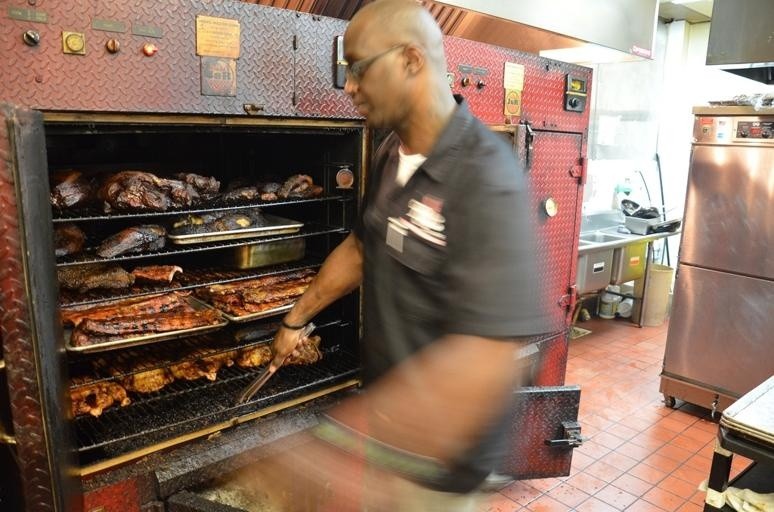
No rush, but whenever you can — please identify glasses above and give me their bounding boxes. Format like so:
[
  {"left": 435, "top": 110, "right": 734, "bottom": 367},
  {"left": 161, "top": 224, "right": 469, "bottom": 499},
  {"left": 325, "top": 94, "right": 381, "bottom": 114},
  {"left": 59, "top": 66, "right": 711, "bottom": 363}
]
[{"left": 343, "top": 44, "right": 410, "bottom": 79}]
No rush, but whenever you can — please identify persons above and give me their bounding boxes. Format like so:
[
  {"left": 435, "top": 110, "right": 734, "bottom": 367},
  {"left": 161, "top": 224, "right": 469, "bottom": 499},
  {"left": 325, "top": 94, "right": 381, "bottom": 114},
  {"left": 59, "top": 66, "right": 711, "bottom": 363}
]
[{"left": 244, "top": 1, "right": 555, "bottom": 512}]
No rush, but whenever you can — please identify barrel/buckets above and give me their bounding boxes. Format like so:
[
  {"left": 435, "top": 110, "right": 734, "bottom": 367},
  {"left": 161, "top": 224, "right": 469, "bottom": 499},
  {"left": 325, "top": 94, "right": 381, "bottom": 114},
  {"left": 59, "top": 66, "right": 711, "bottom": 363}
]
[
  {"left": 598, "top": 285, "right": 620, "bottom": 319},
  {"left": 631, "top": 264, "right": 672, "bottom": 328}
]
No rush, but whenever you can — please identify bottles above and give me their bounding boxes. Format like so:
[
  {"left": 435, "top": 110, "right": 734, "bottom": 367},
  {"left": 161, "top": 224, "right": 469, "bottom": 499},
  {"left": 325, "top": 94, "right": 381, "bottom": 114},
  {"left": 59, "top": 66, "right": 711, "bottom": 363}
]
[
  {"left": 598, "top": 280, "right": 620, "bottom": 319},
  {"left": 581, "top": 308, "right": 590, "bottom": 323}
]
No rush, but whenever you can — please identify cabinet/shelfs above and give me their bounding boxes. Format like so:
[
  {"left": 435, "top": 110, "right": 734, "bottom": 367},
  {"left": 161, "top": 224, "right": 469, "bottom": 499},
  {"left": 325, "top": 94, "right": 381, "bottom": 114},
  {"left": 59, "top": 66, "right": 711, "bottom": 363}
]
[{"left": 703, "top": 427, "right": 774, "bottom": 512}]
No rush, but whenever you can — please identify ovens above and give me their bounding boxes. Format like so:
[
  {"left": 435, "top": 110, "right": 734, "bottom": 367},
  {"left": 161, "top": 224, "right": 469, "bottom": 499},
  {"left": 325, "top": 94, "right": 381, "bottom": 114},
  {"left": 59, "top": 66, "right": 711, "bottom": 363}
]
[{"left": 2, "top": 2, "right": 594, "bottom": 510}]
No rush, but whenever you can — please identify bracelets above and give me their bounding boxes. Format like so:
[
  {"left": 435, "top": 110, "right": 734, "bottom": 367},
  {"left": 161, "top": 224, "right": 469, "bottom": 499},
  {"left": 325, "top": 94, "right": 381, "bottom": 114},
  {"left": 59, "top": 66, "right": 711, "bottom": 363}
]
[{"left": 282, "top": 319, "right": 308, "bottom": 330}]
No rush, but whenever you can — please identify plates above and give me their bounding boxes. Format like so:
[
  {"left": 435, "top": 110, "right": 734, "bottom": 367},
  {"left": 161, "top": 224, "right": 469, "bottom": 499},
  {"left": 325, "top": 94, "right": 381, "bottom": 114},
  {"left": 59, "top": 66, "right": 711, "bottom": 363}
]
[
  {"left": 166, "top": 214, "right": 305, "bottom": 246},
  {"left": 220, "top": 301, "right": 300, "bottom": 326},
  {"left": 63, "top": 293, "right": 228, "bottom": 352}
]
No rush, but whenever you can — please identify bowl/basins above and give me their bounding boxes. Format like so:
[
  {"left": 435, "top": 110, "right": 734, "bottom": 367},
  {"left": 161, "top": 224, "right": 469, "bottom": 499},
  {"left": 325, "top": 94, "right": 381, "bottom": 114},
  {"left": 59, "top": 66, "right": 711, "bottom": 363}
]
[{"left": 620, "top": 199, "right": 644, "bottom": 218}]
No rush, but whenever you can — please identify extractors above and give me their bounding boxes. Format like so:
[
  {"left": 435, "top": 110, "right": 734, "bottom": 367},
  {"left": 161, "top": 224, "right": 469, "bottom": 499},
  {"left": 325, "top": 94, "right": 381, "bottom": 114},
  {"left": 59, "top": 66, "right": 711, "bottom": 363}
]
[{"left": 443, "top": 1, "right": 666, "bottom": 60}]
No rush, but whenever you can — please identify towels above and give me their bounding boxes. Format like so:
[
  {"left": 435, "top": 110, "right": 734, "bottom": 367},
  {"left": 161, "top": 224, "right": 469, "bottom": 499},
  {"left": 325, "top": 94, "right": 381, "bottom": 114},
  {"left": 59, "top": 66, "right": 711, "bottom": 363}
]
[{"left": 698, "top": 478, "right": 774, "bottom": 511}]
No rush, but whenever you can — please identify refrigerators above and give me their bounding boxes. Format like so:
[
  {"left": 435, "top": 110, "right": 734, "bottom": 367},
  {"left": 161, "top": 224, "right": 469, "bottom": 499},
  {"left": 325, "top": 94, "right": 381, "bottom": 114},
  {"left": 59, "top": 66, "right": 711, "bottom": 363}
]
[{"left": 660, "top": 106, "right": 773, "bottom": 415}]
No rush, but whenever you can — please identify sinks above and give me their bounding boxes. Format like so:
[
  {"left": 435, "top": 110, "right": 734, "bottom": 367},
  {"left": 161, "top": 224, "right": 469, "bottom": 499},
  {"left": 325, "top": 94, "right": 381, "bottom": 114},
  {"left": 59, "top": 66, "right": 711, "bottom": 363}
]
[
  {"left": 576, "top": 241, "right": 615, "bottom": 295},
  {"left": 580, "top": 233, "right": 647, "bottom": 283}
]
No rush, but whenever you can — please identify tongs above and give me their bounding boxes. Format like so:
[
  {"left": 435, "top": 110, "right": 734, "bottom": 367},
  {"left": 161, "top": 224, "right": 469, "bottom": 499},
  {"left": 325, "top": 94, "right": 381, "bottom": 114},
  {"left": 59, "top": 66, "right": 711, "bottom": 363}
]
[{"left": 234, "top": 322, "right": 317, "bottom": 406}]
